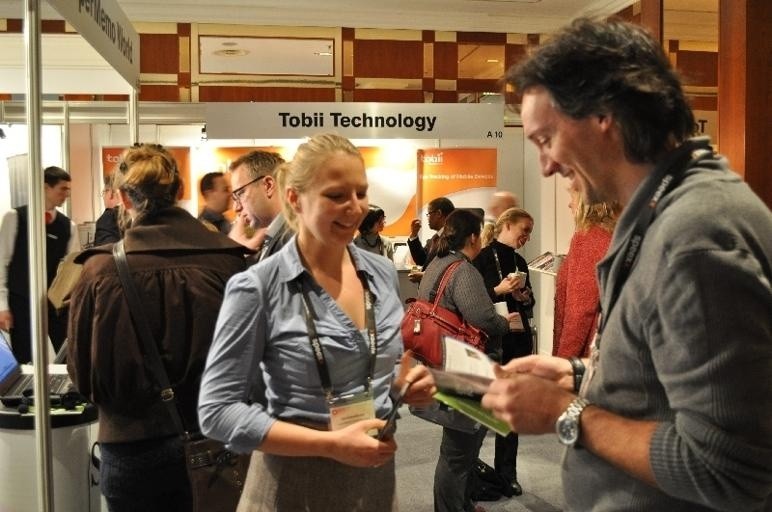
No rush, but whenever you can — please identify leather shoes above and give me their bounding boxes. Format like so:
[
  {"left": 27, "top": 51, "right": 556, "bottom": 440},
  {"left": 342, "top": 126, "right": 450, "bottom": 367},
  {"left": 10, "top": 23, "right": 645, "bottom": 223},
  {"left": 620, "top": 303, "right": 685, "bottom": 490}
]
[{"left": 505, "top": 480, "right": 523, "bottom": 494}]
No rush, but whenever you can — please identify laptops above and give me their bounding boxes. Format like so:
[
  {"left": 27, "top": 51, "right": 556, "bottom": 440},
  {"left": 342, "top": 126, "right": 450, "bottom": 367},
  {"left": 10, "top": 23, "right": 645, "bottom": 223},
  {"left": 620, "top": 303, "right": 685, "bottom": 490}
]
[{"left": 0, "top": 326, "right": 72, "bottom": 405}]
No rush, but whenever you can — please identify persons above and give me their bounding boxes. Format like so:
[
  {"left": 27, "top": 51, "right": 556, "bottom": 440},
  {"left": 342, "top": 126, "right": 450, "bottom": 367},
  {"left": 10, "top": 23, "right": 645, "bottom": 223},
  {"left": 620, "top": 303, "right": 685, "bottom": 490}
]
[
  {"left": 90, "top": 169, "right": 132, "bottom": 248},
  {"left": 195, "top": 171, "right": 521, "bottom": 301},
  {"left": 473, "top": 206, "right": 537, "bottom": 504},
  {"left": 65, "top": 143, "right": 268, "bottom": 512},
  {"left": 479, "top": 12, "right": 771, "bottom": 510},
  {"left": 0, "top": 165, "right": 79, "bottom": 365},
  {"left": 415, "top": 207, "right": 522, "bottom": 511},
  {"left": 195, "top": 132, "right": 439, "bottom": 512},
  {"left": 551, "top": 173, "right": 619, "bottom": 361},
  {"left": 227, "top": 149, "right": 297, "bottom": 267}
]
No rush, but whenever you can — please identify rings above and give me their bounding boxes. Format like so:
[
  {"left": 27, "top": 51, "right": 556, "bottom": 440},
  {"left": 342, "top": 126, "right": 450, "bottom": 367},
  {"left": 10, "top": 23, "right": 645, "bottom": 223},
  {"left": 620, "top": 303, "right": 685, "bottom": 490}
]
[{"left": 372, "top": 464, "right": 379, "bottom": 468}]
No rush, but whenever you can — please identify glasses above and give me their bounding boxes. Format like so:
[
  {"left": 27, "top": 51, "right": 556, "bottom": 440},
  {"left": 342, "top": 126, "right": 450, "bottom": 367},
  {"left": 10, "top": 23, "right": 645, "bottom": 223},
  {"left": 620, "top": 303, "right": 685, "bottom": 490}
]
[{"left": 231, "top": 177, "right": 266, "bottom": 201}]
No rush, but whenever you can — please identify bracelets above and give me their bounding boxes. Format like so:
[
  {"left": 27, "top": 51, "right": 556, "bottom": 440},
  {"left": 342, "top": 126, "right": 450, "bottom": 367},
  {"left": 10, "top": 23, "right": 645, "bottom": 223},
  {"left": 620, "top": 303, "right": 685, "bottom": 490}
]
[{"left": 564, "top": 354, "right": 587, "bottom": 392}]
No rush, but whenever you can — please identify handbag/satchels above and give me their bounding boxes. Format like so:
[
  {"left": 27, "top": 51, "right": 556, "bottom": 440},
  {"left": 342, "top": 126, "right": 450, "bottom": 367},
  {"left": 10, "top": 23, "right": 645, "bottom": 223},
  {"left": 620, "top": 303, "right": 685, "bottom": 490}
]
[
  {"left": 472, "top": 457, "right": 501, "bottom": 502},
  {"left": 409, "top": 399, "right": 481, "bottom": 433},
  {"left": 178, "top": 431, "right": 252, "bottom": 512},
  {"left": 403, "top": 300, "right": 487, "bottom": 368}
]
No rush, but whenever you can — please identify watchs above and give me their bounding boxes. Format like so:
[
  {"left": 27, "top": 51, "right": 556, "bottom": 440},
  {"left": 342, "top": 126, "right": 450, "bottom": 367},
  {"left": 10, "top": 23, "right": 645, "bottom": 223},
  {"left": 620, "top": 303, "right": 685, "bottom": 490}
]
[{"left": 554, "top": 396, "right": 594, "bottom": 452}]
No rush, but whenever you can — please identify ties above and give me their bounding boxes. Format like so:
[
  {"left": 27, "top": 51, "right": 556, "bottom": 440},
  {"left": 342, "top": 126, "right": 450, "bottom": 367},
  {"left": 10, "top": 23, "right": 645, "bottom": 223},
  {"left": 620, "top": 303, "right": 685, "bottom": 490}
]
[{"left": 45, "top": 212, "right": 52, "bottom": 223}]
